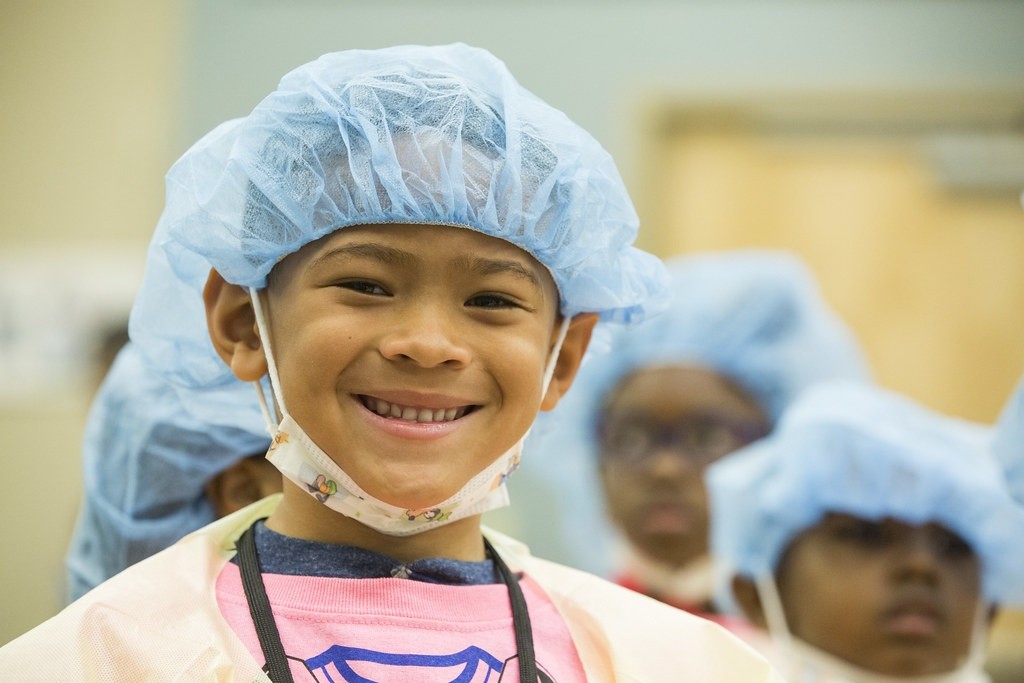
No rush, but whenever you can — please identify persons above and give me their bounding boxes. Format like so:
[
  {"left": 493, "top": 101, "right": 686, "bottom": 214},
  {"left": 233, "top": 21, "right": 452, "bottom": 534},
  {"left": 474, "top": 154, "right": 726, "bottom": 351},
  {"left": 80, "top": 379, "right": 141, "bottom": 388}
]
[
  {"left": 730, "top": 407, "right": 1019, "bottom": 680},
  {"left": 0, "top": 65, "right": 778, "bottom": 683},
  {"left": 83, "top": 358, "right": 293, "bottom": 530},
  {"left": 560, "top": 281, "right": 830, "bottom": 568}
]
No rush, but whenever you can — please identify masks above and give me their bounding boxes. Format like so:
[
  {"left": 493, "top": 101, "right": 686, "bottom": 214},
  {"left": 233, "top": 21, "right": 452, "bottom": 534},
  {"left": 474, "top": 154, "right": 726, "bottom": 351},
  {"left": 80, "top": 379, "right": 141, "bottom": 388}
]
[
  {"left": 245, "top": 285, "right": 571, "bottom": 539},
  {"left": 749, "top": 571, "right": 992, "bottom": 683}
]
